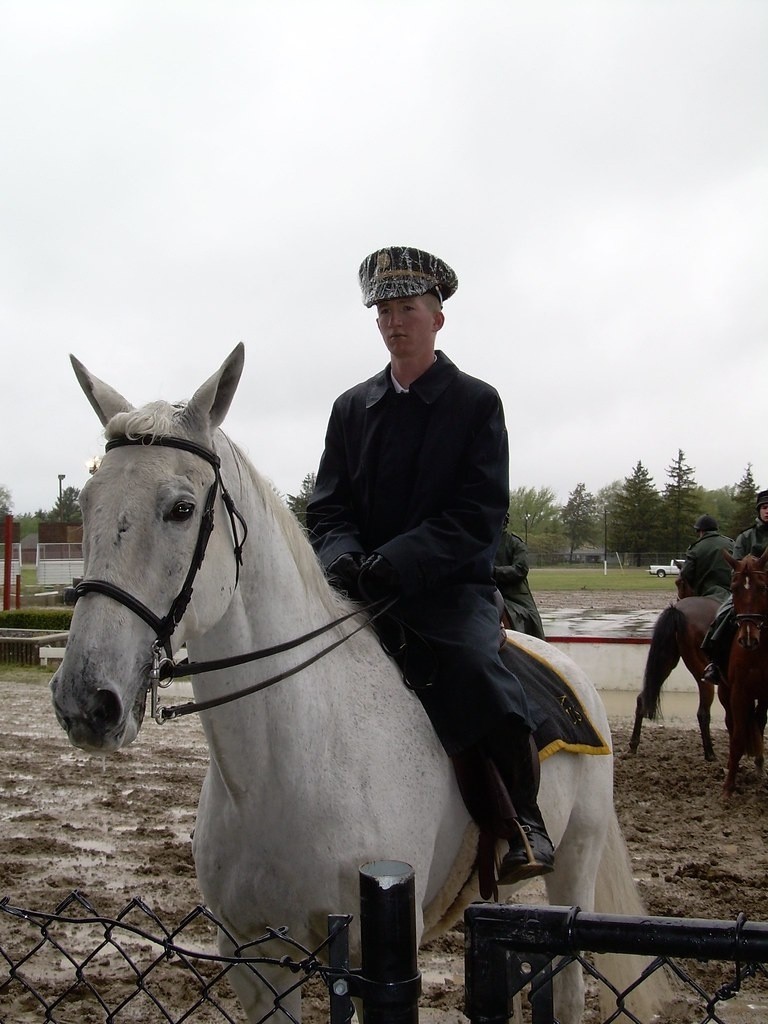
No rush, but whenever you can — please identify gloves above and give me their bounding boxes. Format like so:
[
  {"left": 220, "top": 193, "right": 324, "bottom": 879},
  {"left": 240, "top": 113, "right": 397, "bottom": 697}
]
[{"left": 328, "top": 552, "right": 391, "bottom": 595}]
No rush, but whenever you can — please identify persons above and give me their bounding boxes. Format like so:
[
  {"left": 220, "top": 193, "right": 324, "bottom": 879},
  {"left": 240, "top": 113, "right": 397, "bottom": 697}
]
[
  {"left": 305, "top": 247, "right": 557, "bottom": 884},
  {"left": 680, "top": 490, "right": 768, "bottom": 685}
]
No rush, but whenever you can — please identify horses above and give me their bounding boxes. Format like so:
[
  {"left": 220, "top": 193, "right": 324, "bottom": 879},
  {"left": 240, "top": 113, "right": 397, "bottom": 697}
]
[
  {"left": 48, "top": 341, "right": 688, "bottom": 1024},
  {"left": 624, "top": 544, "right": 768, "bottom": 801}
]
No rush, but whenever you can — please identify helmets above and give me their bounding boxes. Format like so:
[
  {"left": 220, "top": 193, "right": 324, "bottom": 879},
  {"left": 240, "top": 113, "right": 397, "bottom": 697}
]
[
  {"left": 756, "top": 490, "right": 768, "bottom": 510},
  {"left": 693, "top": 514, "right": 720, "bottom": 532}
]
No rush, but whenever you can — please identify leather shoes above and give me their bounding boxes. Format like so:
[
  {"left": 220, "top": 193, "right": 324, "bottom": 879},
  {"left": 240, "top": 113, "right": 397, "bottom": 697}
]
[
  {"left": 501, "top": 833, "right": 559, "bottom": 873},
  {"left": 704, "top": 666, "right": 719, "bottom": 683}
]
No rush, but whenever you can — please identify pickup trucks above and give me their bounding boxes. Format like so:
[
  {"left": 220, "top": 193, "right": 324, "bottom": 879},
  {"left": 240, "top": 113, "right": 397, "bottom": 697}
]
[{"left": 649, "top": 559, "right": 686, "bottom": 578}]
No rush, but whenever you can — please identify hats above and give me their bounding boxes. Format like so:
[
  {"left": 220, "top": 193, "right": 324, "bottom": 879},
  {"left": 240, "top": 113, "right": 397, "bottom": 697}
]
[{"left": 358, "top": 246, "right": 458, "bottom": 308}]
